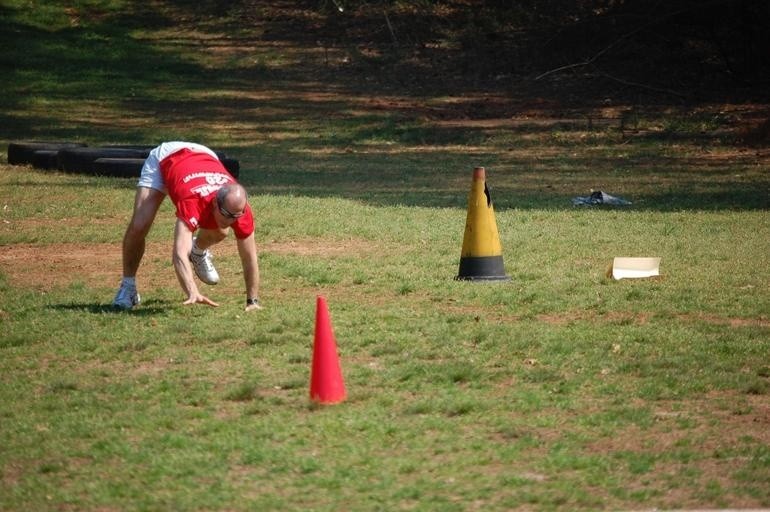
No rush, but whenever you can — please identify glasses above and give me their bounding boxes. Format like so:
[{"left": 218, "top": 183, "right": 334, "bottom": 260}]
[{"left": 217, "top": 199, "right": 245, "bottom": 218}]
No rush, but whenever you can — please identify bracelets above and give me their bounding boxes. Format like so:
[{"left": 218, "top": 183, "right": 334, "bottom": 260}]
[{"left": 247, "top": 298, "right": 257, "bottom": 304}]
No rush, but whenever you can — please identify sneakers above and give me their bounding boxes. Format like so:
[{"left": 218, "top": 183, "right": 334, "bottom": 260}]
[
  {"left": 189, "top": 237, "right": 219, "bottom": 285},
  {"left": 113, "top": 280, "right": 140, "bottom": 308}
]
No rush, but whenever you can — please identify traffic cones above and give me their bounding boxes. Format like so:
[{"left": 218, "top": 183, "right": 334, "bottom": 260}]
[
  {"left": 454, "top": 166, "right": 511, "bottom": 281},
  {"left": 309, "top": 295, "right": 347, "bottom": 404}
]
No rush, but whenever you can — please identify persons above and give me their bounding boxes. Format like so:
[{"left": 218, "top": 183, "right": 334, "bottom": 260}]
[{"left": 112, "top": 141, "right": 264, "bottom": 311}]
